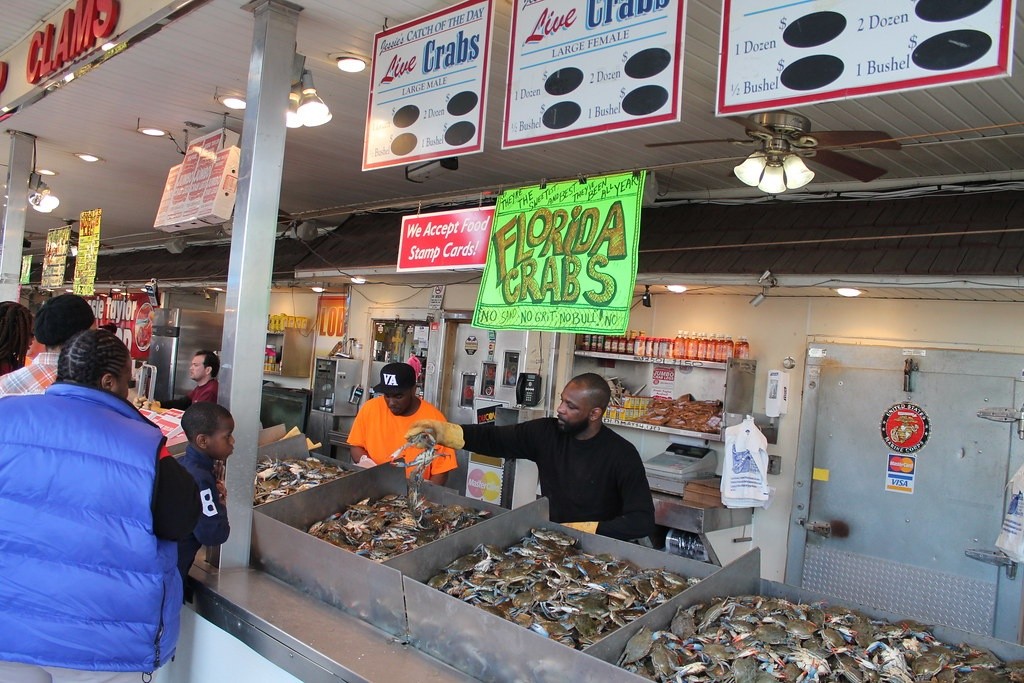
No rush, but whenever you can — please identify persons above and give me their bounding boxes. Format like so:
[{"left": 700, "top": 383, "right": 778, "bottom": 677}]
[
  {"left": 346, "top": 363, "right": 459, "bottom": 485},
  {"left": 0, "top": 301, "right": 34, "bottom": 375},
  {"left": 0, "top": 330, "right": 202, "bottom": 683},
  {"left": 175, "top": 403, "right": 235, "bottom": 579},
  {"left": 99, "top": 323, "right": 117, "bottom": 334},
  {"left": 0, "top": 295, "right": 96, "bottom": 395},
  {"left": 405, "top": 372, "right": 656, "bottom": 542},
  {"left": 133, "top": 350, "right": 220, "bottom": 410}
]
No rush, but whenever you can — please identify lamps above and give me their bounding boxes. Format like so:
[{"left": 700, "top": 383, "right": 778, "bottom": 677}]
[
  {"left": 28, "top": 185, "right": 59, "bottom": 214},
  {"left": 734, "top": 138, "right": 815, "bottom": 194},
  {"left": 286, "top": 69, "right": 333, "bottom": 129}
]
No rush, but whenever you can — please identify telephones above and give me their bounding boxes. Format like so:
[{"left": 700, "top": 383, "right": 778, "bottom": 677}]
[{"left": 516, "top": 372, "right": 542, "bottom": 406}]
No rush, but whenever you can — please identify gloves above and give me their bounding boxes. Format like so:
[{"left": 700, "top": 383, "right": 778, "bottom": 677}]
[
  {"left": 404, "top": 419, "right": 466, "bottom": 450},
  {"left": 560, "top": 521, "right": 599, "bottom": 533}
]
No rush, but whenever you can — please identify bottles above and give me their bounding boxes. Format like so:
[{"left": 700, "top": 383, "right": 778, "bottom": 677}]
[
  {"left": 355, "top": 338, "right": 363, "bottom": 360},
  {"left": 582, "top": 329, "right": 750, "bottom": 363}
]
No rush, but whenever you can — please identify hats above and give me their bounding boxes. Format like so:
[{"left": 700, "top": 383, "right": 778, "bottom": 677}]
[
  {"left": 373, "top": 362, "right": 416, "bottom": 394},
  {"left": 33, "top": 294, "right": 94, "bottom": 344},
  {"left": 99, "top": 323, "right": 116, "bottom": 334}
]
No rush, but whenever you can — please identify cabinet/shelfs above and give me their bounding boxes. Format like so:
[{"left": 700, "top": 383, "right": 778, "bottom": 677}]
[{"left": 573, "top": 351, "right": 757, "bottom": 441}]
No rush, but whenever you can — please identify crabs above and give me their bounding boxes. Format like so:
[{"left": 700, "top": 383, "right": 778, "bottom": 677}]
[{"left": 216, "top": 432, "right": 1024, "bottom": 683}]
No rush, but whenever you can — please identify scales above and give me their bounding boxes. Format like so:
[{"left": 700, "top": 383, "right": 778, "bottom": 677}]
[{"left": 643, "top": 443, "right": 718, "bottom": 496}]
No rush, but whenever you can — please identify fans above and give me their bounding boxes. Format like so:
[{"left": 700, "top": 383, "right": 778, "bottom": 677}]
[{"left": 644, "top": 111, "right": 901, "bottom": 196}]
